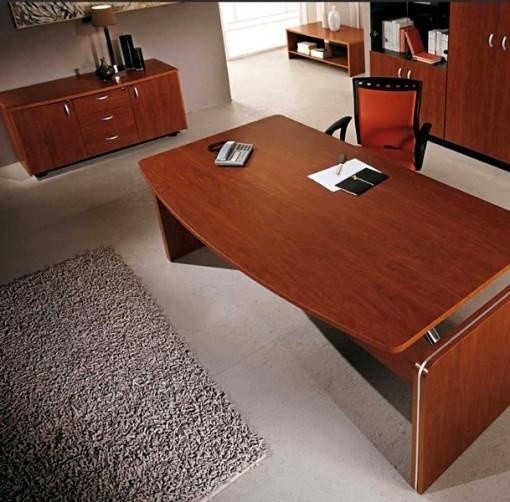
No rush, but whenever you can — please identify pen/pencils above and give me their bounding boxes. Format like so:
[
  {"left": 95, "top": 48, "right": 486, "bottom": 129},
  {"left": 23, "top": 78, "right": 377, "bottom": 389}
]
[{"left": 336, "top": 164, "right": 343, "bottom": 175}]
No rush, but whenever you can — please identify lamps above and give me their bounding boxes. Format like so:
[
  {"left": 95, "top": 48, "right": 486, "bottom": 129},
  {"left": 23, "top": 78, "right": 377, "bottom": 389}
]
[{"left": 92, "top": 4, "right": 127, "bottom": 79}]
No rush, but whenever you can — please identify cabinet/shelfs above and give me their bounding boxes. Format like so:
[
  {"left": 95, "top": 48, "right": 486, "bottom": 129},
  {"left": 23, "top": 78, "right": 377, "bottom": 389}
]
[
  {"left": 286, "top": 29, "right": 365, "bottom": 77},
  {"left": 370, "top": 0, "right": 450, "bottom": 147},
  {"left": 0, "top": 70, "right": 188, "bottom": 178},
  {"left": 443, "top": 0, "right": 510, "bottom": 172}
]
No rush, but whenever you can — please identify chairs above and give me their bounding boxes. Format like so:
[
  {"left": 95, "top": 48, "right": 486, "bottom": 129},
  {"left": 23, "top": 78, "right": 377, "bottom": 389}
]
[{"left": 325, "top": 77, "right": 432, "bottom": 175}]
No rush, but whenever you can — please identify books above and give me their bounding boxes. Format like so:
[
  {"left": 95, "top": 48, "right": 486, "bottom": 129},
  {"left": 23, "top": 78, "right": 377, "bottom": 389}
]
[
  {"left": 335, "top": 167, "right": 389, "bottom": 196},
  {"left": 381, "top": 17, "right": 449, "bottom": 65}
]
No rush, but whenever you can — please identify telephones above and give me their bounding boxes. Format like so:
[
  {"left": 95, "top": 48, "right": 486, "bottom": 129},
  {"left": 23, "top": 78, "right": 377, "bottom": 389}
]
[{"left": 214, "top": 139, "right": 254, "bottom": 167}]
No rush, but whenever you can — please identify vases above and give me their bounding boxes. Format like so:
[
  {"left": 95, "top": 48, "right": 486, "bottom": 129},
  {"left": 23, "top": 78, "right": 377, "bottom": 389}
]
[{"left": 328, "top": 5, "right": 341, "bottom": 31}]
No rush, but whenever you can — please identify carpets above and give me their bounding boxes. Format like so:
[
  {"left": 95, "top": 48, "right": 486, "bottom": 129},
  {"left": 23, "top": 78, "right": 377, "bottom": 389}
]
[{"left": 0, "top": 244, "right": 268, "bottom": 502}]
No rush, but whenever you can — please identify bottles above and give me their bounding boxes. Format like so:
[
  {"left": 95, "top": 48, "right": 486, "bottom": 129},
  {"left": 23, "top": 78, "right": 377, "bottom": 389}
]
[
  {"left": 119, "top": 34, "right": 136, "bottom": 71},
  {"left": 131, "top": 47, "right": 146, "bottom": 72},
  {"left": 329, "top": 7, "right": 340, "bottom": 31}
]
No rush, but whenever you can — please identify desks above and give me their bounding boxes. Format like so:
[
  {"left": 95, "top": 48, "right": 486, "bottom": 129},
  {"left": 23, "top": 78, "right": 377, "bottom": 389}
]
[{"left": 138, "top": 114, "right": 510, "bottom": 495}]
